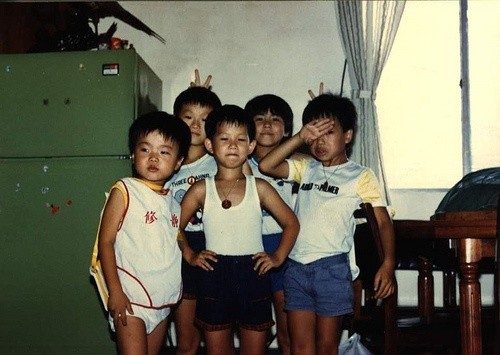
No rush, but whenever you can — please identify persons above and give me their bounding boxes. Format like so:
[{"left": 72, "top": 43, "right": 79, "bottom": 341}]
[
  {"left": 97, "top": 111, "right": 192, "bottom": 355},
  {"left": 163, "top": 86, "right": 222, "bottom": 355},
  {"left": 176, "top": 105, "right": 301, "bottom": 355},
  {"left": 188, "top": 68, "right": 324, "bottom": 355},
  {"left": 258, "top": 93, "right": 396, "bottom": 355}
]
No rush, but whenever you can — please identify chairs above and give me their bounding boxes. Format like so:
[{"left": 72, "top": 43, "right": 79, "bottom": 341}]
[{"left": 349, "top": 220, "right": 462, "bottom": 355}]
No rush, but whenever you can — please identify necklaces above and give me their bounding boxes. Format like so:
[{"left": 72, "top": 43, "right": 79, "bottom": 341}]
[
  {"left": 321, "top": 156, "right": 347, "bottom": 190},
  {"left": 184, "top": 152, "right": 210, "bottom": 185},
  {"left": 215, "top": 173, "right": 243, "bottom": 209}
]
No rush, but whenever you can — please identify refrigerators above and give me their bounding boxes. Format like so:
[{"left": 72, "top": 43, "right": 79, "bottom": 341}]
[{"left": 1, "top": 51, "right": 162, "bottom": 355}]
